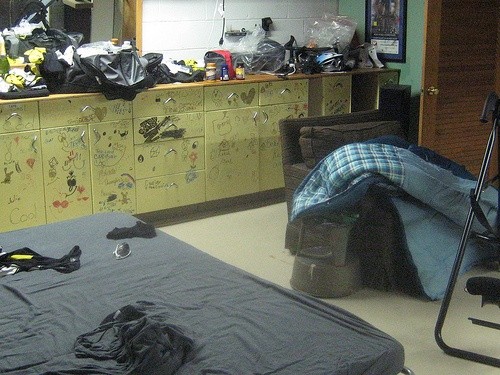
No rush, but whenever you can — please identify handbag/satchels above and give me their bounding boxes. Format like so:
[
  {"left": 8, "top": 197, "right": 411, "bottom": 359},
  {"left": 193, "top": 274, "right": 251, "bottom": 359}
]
[
  {"left": 42, "top": 40, "right": 161, "bottom": 98},
  {"left": 5, "top": 31, "right": 86, "bottom": 60},
  {"left": 204, "top": 50, "right": 234, "bottom": 79}
]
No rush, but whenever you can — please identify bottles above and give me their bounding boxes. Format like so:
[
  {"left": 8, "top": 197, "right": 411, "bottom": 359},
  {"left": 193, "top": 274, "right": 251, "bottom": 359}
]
[
  {"left": 206, "top": 63, "right": 217, "bottom": 82},
  {"left": 236, "top": 62, "right": 245, "bottom": 80},
  {"left": 219, "top": 64, "right": 229, "bottom": 81}
]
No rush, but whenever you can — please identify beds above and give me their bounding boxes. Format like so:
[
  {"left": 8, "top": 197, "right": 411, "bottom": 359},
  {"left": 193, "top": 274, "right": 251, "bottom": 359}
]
[{"left": 0, "top": 213, "right": 415, "bottom": 375}]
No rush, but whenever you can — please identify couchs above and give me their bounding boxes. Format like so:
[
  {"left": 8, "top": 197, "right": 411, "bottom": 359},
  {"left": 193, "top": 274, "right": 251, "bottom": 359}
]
[{"left": 278, "top": 109, "right": 409, "bottom": 290}]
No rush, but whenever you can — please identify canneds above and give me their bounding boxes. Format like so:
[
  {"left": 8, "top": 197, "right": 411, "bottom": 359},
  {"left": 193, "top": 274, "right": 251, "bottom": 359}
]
[{"left": 206, "top": 62, "right": 245, "bottom": 83}]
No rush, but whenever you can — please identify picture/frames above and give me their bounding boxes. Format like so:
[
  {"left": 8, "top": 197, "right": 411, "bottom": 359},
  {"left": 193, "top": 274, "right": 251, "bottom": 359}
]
[{"left": 364, "top": 0, "right": 408, "bottom": 63}]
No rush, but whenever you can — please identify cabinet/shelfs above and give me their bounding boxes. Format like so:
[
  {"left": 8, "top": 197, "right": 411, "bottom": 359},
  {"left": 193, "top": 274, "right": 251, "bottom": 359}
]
[{"left": 0, "top": 71, "right": 399, "bottom": 231}]
